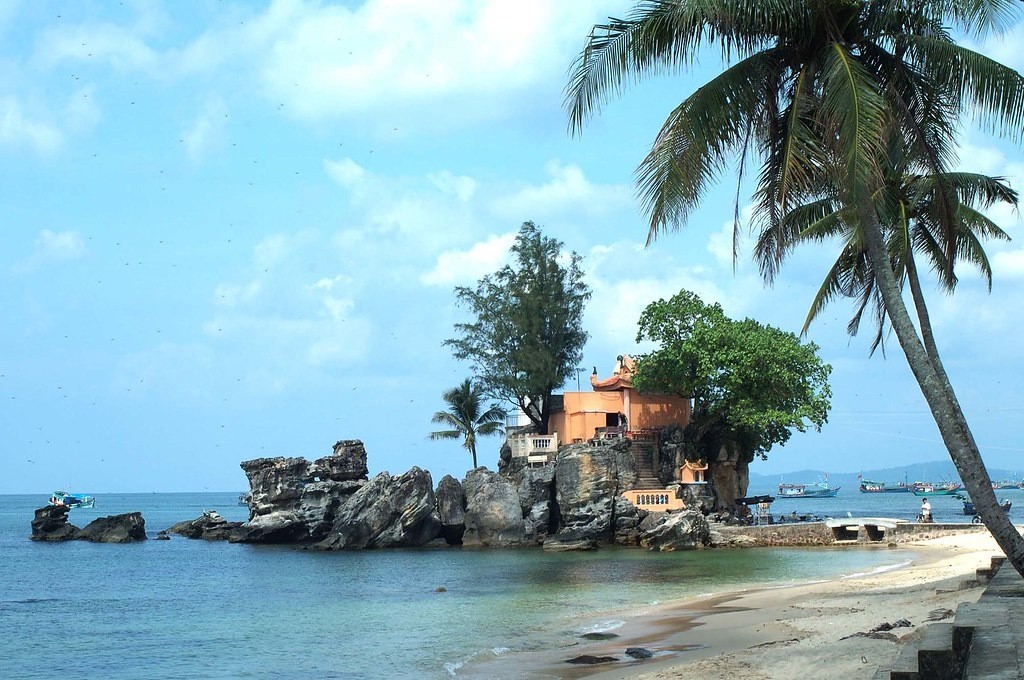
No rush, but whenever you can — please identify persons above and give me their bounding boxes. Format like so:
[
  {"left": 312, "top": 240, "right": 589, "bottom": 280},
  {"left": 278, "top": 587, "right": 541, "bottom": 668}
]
[
  {"left": 921, "top": 498, "right": 931, "bottom": 523},
  {"left": 617, "top": 411, "right": 628, "bottom": 432}
]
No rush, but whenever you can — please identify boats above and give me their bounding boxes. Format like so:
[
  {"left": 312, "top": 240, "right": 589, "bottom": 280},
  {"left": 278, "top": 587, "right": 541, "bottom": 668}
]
[
  {"left": 936, "top": 473, "right": 1024, "bottom": 490},
  {"left": 776, "top": 473, "right": 842, "bottom": 498},
  {"left": 912, "top": 483, "right": 961, "bottom": 495},
  {"left": 48, "top": 490, "right": 95, "bottom": 509},
  {"left": 857, "top": 471, "right": 909, "bottom": 493},
  {"left": 963, "top": 498, "right": 1012, "bottom": 517}
]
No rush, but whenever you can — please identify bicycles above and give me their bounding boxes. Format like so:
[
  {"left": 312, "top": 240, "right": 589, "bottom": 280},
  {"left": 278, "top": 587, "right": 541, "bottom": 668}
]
[{"left": 970, "top": 504, "right": 982, "bottom": 523}]
